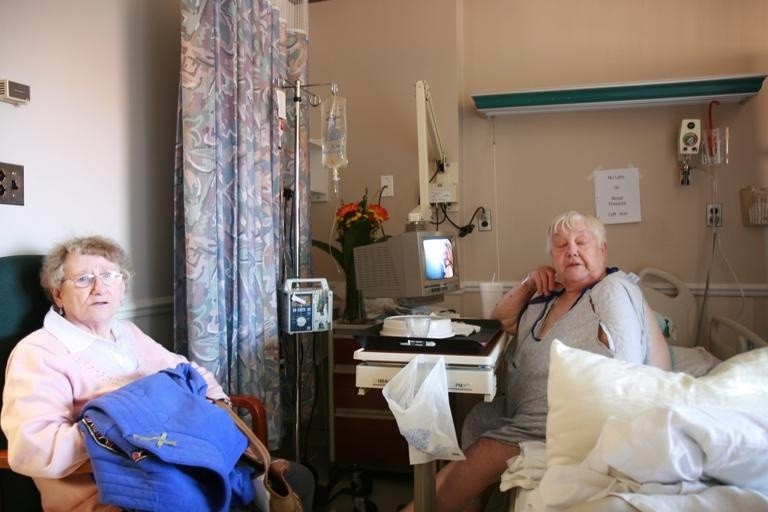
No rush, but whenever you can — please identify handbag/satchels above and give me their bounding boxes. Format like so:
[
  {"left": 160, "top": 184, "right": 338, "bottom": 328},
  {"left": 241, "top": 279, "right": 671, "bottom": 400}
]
[{"left": 235, "top": 453, "right": 305, "bottom": 512}]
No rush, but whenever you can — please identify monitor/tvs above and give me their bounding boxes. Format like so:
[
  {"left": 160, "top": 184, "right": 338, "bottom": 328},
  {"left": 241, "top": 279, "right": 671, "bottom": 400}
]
[{"left": 352, "top": 230, "right": 459, "bottom": 309}]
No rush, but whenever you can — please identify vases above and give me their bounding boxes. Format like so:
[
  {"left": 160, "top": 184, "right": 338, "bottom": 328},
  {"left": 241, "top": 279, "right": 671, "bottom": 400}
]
[{"left": 342, "top": 271, "right": 367, "bottom": 322}]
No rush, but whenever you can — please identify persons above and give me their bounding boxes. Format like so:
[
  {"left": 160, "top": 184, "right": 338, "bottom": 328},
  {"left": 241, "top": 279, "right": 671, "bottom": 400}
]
[
  {"left": 0, "top": 235, "right": 315, "bottom": 512},
  {"left": 397, "top": 210, "right": 672, "bottom": 512}
]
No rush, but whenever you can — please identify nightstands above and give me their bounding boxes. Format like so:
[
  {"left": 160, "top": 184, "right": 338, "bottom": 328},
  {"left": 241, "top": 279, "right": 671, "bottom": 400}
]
[{"left": 327, "top": 330, "right": 415, "bottom": 470}]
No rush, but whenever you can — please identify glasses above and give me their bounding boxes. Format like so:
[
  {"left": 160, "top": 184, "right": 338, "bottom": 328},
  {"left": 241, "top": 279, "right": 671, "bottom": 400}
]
[{"left": 62, "top": 271, "right": 124, "bottom": 286}]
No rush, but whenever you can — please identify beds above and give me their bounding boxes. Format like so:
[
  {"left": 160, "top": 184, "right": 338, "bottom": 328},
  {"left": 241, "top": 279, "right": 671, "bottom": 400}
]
[{"left": 499, "top": 265, "right": 768, "bottom": 512}]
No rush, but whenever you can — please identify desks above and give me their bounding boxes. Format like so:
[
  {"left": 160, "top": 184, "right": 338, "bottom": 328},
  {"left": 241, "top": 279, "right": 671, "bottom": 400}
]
[{"left": 352, "top": 318, "right": 507, "bottom": 512}]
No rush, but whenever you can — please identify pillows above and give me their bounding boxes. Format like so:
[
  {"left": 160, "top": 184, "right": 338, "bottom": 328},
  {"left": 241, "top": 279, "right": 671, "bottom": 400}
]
[{"left": 544, "top": 336, "right": 768, "bottom": 492}]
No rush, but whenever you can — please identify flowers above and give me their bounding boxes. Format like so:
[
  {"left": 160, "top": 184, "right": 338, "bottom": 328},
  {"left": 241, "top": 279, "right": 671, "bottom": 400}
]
[{"left": 313, "top": 188, "right": 389, "bottom": 322}]
